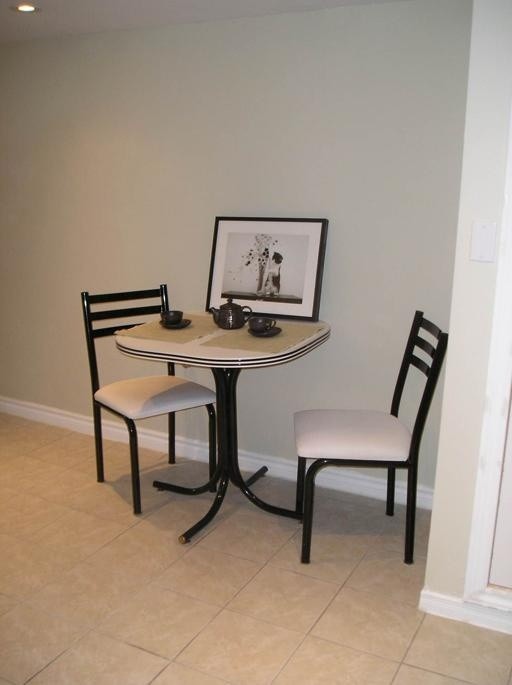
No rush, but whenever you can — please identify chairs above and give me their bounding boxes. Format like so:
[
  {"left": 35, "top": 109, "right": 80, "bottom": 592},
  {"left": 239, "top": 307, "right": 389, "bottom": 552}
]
[
  {"left": 82, "top": 284, "right": 218, "bottom": 514},
  {"left": 294, "top": 311, "right": 448, "bottom": 564}
]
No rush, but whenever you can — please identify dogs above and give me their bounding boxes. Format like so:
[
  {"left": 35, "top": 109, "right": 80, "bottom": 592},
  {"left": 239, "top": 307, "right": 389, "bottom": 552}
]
[{"left": 256, "top": 250, "right": 283, "bottom": 297}]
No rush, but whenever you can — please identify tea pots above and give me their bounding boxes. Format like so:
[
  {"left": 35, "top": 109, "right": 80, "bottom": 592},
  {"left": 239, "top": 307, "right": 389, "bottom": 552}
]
[{"left": 210, "top": 297, "right": 253, "bottom": 329}]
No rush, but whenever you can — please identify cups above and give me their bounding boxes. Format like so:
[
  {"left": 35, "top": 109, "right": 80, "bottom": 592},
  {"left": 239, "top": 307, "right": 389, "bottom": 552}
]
[
  {"left": 161, "top": 311, "right": 183, "bottom": 323},
  {"left": 249, "top": 318, "right": 276, "bottom": 332}
]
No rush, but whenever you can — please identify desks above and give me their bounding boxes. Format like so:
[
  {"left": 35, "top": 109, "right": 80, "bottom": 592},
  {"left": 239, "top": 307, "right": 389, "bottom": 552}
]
[{"left": 115, "top": 314, "right": 331, "bottom": 544}]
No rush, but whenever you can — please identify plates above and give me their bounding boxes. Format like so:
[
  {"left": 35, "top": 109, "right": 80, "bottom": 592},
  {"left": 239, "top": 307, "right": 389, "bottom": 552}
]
[
  {"left": 159, "top": 320, "right": 191, "bottom": 329},
  {"left": 248, "top": 328, "right": 281, "bottom": 337}
]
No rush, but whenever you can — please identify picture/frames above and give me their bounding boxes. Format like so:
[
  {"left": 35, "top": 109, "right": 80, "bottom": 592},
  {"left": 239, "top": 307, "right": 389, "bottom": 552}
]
[{"left": 206, "top": 216, "right": 329, "bottom": 322}]
